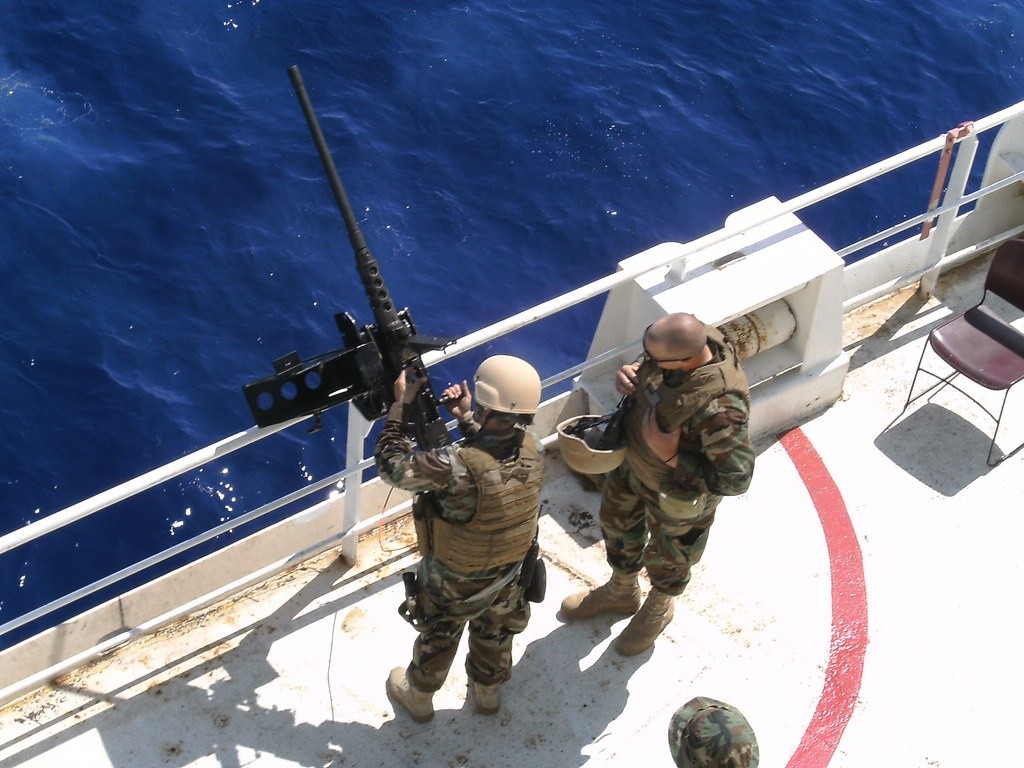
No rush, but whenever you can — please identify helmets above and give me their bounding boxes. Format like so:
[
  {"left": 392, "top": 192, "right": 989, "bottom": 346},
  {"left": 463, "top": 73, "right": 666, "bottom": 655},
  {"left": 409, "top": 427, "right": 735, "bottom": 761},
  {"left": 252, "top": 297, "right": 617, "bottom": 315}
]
[
  {"left": 557, "top": 414, "right": 627, "bottom": 473},
  {"left": 472, "top": 354, "right": 540, "bottom": 413}
]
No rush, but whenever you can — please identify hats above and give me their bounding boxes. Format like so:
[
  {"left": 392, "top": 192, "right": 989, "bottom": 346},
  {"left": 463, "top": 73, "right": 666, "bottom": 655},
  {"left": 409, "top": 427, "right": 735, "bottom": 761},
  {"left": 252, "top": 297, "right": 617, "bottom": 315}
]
[{"left": 668, "top": 696, "right": 759, "bottom": 768}]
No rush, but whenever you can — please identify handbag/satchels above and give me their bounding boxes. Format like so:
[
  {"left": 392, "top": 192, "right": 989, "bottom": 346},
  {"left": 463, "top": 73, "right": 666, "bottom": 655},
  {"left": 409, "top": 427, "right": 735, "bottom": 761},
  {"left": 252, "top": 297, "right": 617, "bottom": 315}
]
[{"left": 525, "top": 556, "right": 546, "bottom": 603}]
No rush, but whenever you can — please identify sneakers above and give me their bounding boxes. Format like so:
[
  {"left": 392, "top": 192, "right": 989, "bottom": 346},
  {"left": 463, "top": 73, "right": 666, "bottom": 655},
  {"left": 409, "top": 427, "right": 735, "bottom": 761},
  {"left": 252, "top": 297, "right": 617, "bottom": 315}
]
[
  {"left": 473, "top": 680, "right": 500, "bottom": 715},
  {"left": 389, "top": 667, "right": 434, "bottom": 723},
  {"left": 615, "top": 587, "right": 675, "bottom": 657},
  {"left": 561, "top": 573, "right": 641, "bottom": 620}
]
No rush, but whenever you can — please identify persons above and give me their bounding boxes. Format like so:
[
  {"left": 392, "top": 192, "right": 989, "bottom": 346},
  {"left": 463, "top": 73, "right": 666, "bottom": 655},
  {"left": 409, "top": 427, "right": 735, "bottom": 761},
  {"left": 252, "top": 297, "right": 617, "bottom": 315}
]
[
  {"left": 668, "top": 696, "right": 760, "bottom": 768},
  {"left": 561, "top": 313, "right": 755, "bottom": 656},
  {"left": 375, "top": 354, "right": 541, "bottom": 722}
]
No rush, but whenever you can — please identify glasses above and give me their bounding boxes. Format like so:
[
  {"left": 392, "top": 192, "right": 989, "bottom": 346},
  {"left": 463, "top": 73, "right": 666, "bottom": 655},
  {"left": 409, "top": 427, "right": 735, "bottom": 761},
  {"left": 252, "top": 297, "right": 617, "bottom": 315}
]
[{"left": 643, "top": 324, "right": 693, "bottom": 366}]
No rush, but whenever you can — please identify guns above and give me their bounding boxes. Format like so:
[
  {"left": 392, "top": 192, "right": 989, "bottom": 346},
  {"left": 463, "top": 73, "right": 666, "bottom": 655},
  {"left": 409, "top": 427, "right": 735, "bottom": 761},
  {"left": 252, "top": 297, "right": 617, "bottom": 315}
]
[{"left": 245, "top": 63, "right": 465, "bottom": 450}]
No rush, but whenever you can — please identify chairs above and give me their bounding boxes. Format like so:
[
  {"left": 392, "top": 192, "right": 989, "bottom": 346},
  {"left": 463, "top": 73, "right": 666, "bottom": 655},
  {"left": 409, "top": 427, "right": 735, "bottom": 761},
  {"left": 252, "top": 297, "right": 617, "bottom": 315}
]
[{"left": 906, "top": 239, "right": 1024, "bottom": 467}]
